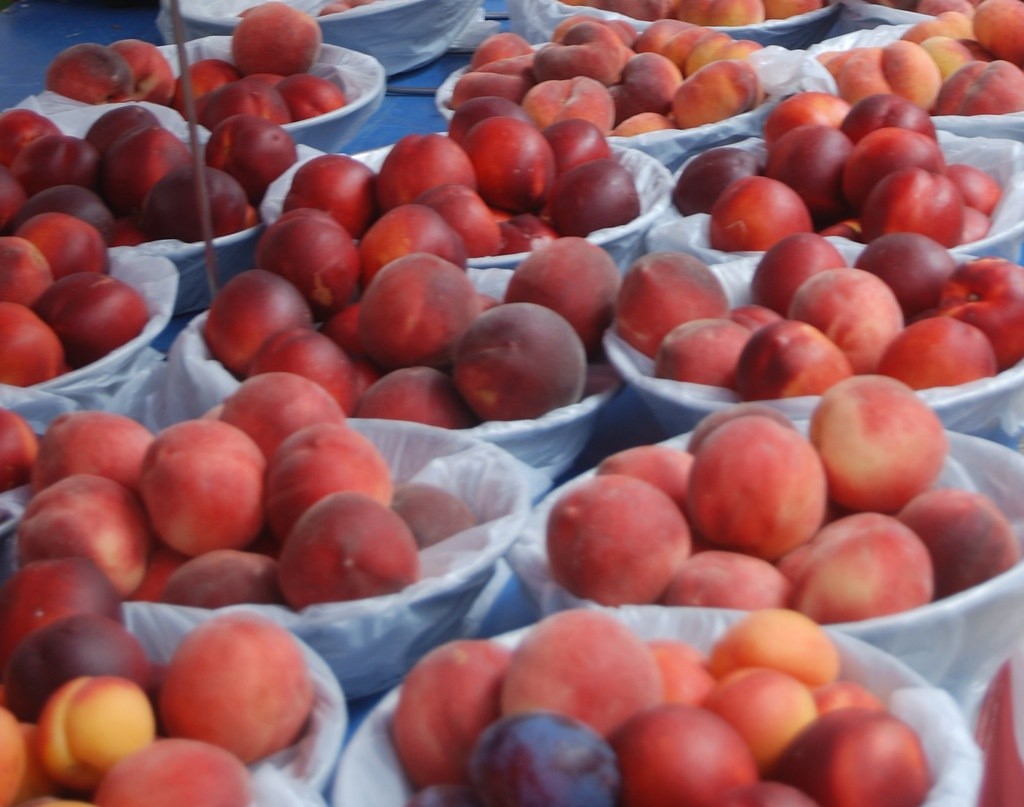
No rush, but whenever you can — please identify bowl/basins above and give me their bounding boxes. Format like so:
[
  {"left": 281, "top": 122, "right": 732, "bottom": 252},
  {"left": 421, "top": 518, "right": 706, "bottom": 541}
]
[{"left": 1, "top": 2, "right": 1024, "bottom": 807}]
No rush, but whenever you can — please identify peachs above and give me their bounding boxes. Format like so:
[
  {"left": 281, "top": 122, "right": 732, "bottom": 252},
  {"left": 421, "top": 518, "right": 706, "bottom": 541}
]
[{"left": 0, "top": 0, "right": 1024, "bottom": 807}]
